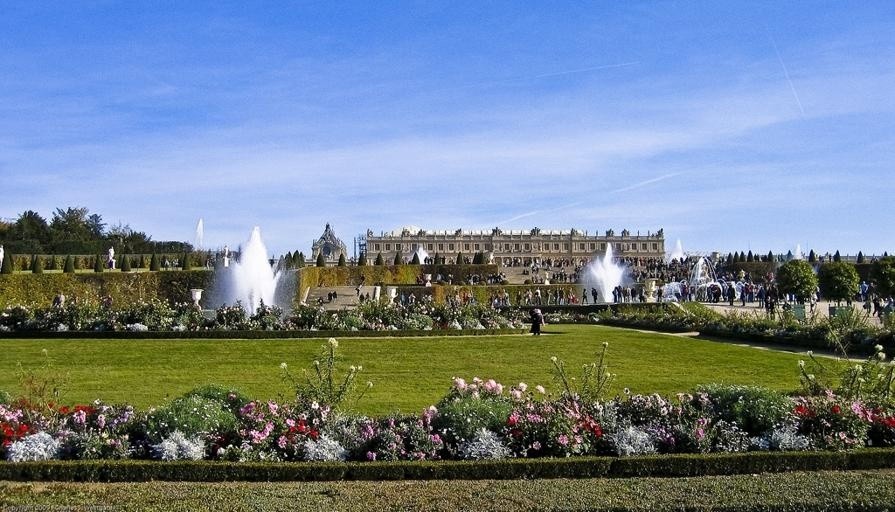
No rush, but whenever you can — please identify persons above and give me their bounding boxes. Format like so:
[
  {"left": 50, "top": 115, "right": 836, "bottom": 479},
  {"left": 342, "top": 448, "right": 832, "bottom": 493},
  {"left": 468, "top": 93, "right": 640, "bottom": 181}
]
[
  {"left": 355, "top": 273, "right": 372, "bottom": 304},
  {"left": 106, "top": 245, "right": 116, "bottom": 270},
  {"left": 327, "top": 290, "right": 337, "bottom": 303},
  {"left": 398, "top": 291, "right": 417, "bottom": 306},
  {"left": 318, "top": 297, "right": 325, "bottom": 307},
  {"left": 350, "top": 256, "right": 355, "bottom": 266},
  {"left": 162, "top": 255, "right": 180, "bottom": 272}
]
